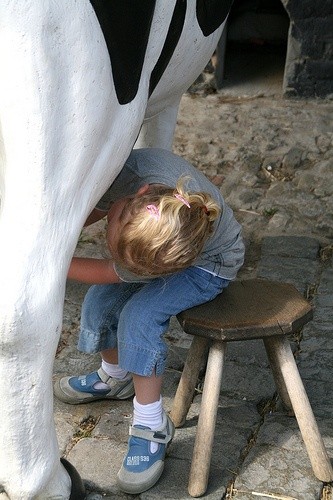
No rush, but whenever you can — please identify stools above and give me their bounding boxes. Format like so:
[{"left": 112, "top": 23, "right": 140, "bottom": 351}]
[{"left": 166, "top": 276, "right": 333, "bottom": 497}]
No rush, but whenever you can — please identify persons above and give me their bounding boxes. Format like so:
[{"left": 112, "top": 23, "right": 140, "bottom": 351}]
[{"left": 51, "top": 149, "right": 246, "bottom": 495}]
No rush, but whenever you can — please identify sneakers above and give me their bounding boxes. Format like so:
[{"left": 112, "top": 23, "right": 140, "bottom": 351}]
[
  {"left": 55, "top": 367, "right": 134, "bottom": 405},
  {"left": 115, "top": 407, "right": 176, "bottom": 495}
]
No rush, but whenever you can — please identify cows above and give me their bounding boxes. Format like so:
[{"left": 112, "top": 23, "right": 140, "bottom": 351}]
[{"left": 2, "top": 0, "right": 230, "bottom": 500}]
